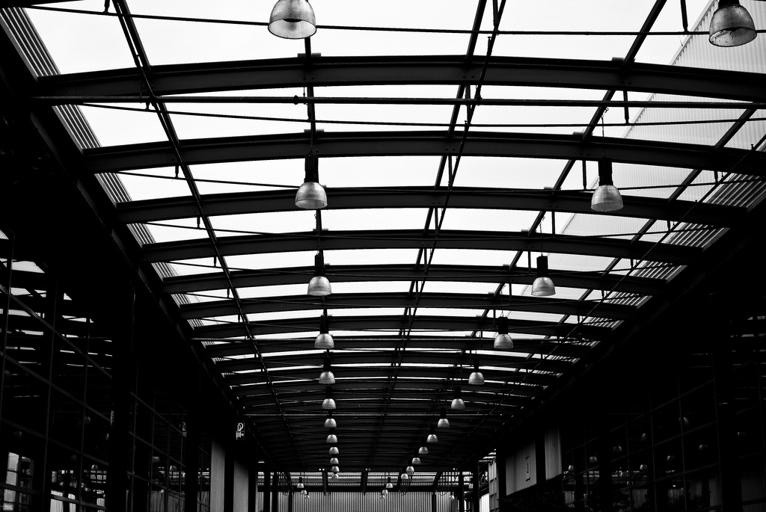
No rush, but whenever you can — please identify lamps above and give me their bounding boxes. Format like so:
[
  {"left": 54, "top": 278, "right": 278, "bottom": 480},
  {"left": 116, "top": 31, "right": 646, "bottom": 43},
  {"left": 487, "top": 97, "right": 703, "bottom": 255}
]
[
  {"left": 590, "top": 157, "right": 623, "bottom": 212},
  {"left": 708, "top": 0, "right": 757, "bottom": 48},
  {"left": 295, "top": 154, "right": 328, "bottom": 209},
  {"left": 267, "top": 0, "right": 316, "bottom": 39},
  {"left": 314, "top": 315, "right": 335, "bottom": 349},
  {"left": 307, "top": 252, "right": 331, "bottom": 296},
  {"left": 318, "top": 363, "right": 335, "bottom": 385},
  {"left": 297, "top": 387, "right": 465, "bottom": 499},
  {"left": 468, "top": 360, "right": 485, "bottom": 385},
  {"left": 532, "top": 249, "right": 555, "bottom": 297},
  {"left": 493, "top": 309, "right": 516, "bottom": 350}
]
